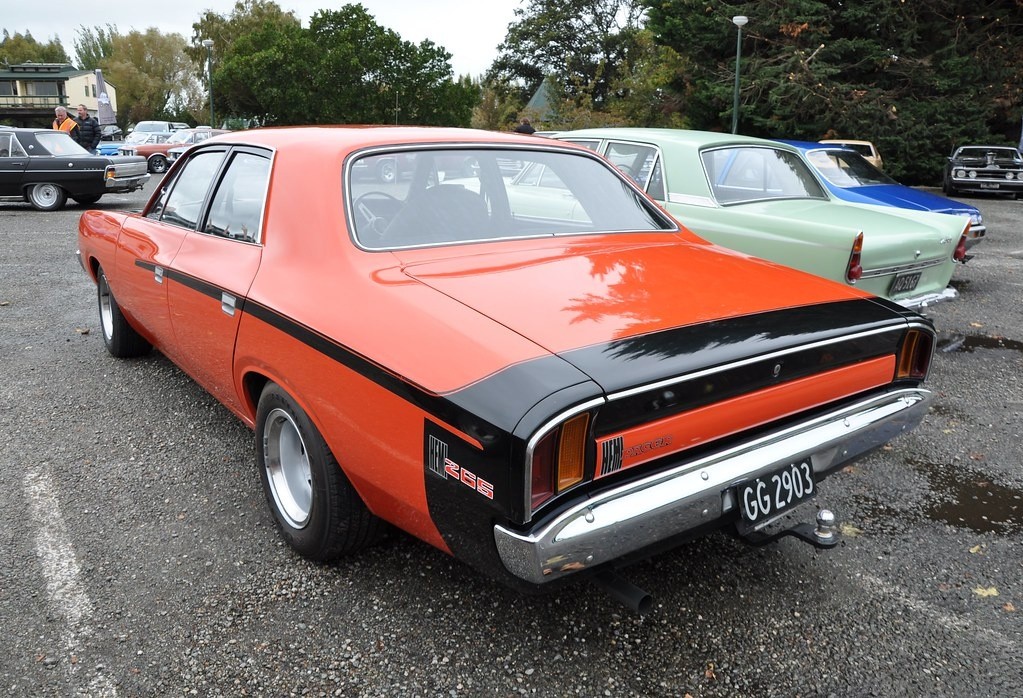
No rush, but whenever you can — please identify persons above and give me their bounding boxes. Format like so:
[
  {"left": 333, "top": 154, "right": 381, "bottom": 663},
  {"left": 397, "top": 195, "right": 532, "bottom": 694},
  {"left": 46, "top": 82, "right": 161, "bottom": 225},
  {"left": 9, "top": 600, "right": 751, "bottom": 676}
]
[
  {"left": 52, "top": 106, "right": 82, "bottom": 143},
  {"left": 513, "top": 118, "right": 536, "bottom": 135},
  {"left": 73, "top": 103, "right": 102, "bottom": 155}
]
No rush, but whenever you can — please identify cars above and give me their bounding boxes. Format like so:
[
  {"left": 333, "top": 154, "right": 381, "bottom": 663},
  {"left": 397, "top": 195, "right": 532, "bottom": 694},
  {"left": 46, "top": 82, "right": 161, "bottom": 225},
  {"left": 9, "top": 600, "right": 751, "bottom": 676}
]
[
  {"left": 76, "top": 123, "right": 935, "bottom": 585},
  {"left": 95, "top": 120, "right": 213, "bottom": 157},
  {"left": 807, "top": 139, "right": 884, "bottom": 173},
  {"left": 434, "top": 129, "right": 969, "bottom": 310},
  {"left": 635, "top": 140, "right": 986, "bottom": 266},
  {"left": 350, "top": 150, "right": 480, "bottom": 184},
  {"left": 117, "top": 129, "right": 232, "bottom": 173},
  {"left": 99, "top": 125, "right": 124, "bottom": 141},
  {"left": 942, "top": 147, "right": 1023, "bottom": 199},
  {"left": 0, "top": 125, "right": 152, "bottom": 212}
]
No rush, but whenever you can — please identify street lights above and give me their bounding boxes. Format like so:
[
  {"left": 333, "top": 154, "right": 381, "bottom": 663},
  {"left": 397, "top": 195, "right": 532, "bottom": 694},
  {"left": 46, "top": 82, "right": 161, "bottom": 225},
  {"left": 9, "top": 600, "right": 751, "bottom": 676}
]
[
  {"left": 733, "top": 16, "right": 749, "bottom": 134},
  {"left": 203, "top": 40, "right": 215, "bottom": 129}
]
